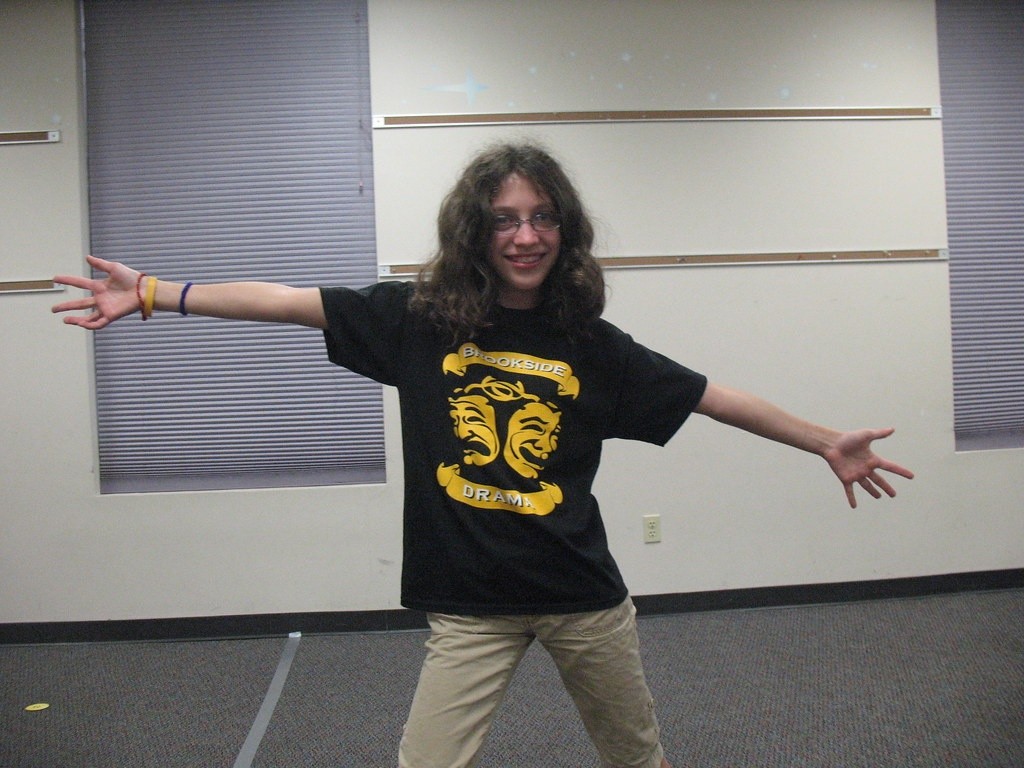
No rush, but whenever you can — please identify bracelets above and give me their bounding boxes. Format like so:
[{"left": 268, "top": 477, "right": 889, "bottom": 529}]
[
  {"left": 137, "top": 273, "right": 147, "bottom": 322},
  {"left": 178, "top": 281, "right": 193, "bottom": 316},
  {"left": 145, "top": 276, "right": 157, "bottom": 318}
]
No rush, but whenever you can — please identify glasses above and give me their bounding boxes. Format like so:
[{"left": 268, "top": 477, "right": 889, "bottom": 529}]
[{"left": 490, "top": 212, "right": 568, "bottom": 234}]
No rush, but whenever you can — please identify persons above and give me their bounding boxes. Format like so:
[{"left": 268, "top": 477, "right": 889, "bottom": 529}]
[{"left": 51, "top": 142, "right": 915, "bottom": 768}]
